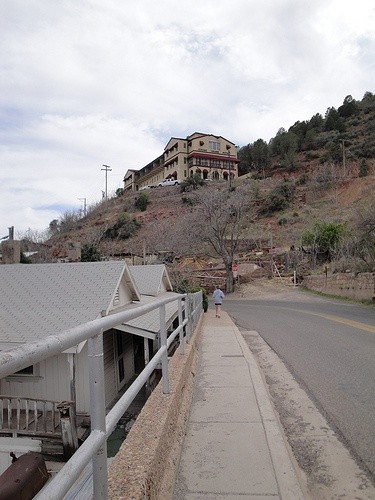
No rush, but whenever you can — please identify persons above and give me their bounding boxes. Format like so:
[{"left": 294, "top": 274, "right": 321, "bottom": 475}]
[{"left": 211, "top": 286, "right": 225, "bottom": 317}]
